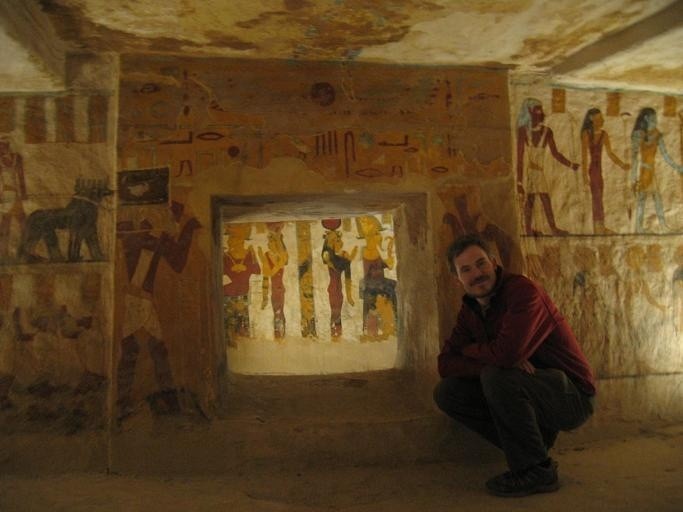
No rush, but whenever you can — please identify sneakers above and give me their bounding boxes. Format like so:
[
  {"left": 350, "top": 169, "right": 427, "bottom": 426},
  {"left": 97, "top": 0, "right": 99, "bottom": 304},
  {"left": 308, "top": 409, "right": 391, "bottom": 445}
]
[{"left": 484, "top": 429, "right": 560, "bottom": 498}]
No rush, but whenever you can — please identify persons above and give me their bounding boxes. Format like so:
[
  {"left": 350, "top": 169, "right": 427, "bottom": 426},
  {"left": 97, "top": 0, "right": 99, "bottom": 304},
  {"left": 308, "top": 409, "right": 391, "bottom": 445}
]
[
  {"left": 515, "top": 97, "right": 683, "bottom": 234},
  {"left": 433, "top": 235, "right": 598, "bottom": 497},
  {"left": 0, "top": 139, "right": 48, "bottom": 264},
  {"left": 223, "top": 222, "right": 397, "bottom": 339}
]
